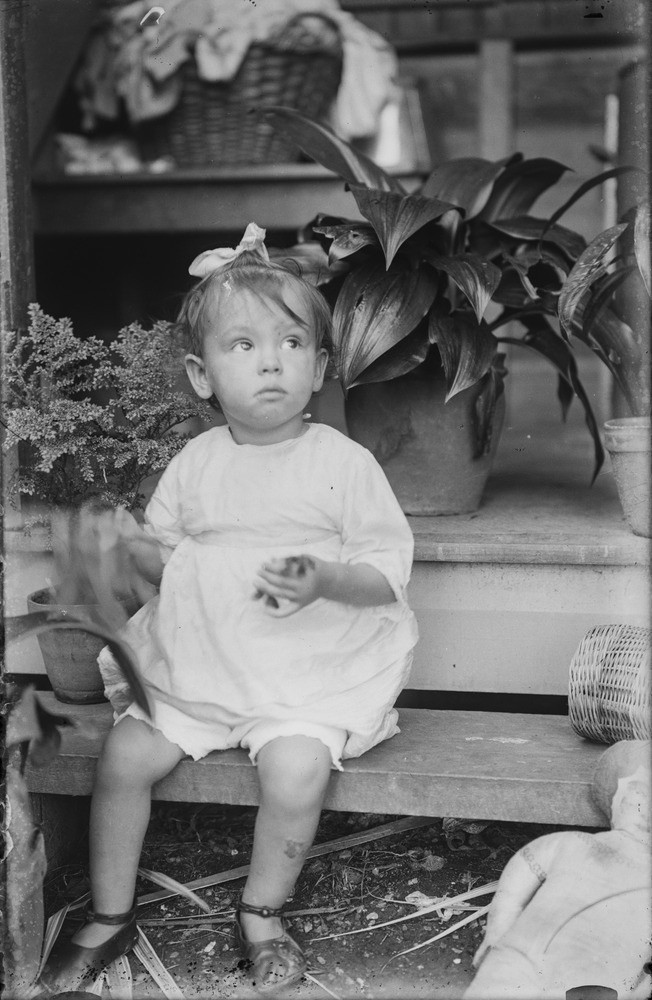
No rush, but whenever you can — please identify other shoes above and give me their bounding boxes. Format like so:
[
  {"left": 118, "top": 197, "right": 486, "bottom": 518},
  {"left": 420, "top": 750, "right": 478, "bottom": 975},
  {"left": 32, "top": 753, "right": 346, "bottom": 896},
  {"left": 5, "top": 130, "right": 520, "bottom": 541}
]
[
  {"left": 234, "top": 896, "right": 307, "bottom": 992},
  {"left": 44, "top": 897, "right": 139, "bottom": 991}
]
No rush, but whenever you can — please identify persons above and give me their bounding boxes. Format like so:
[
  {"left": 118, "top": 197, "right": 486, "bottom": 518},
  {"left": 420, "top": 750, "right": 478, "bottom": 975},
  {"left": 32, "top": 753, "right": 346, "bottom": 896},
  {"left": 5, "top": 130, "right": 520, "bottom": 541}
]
[{"left": 40, "top": 221, "right": 414, "bottom": 995}]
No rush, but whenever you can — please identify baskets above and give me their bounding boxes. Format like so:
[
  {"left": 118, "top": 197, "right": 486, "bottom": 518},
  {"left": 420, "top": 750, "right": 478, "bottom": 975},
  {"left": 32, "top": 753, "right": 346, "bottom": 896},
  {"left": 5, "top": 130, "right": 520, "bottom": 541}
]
[{"left": 569, "top": 626, "right": 649, "bottom": 744}]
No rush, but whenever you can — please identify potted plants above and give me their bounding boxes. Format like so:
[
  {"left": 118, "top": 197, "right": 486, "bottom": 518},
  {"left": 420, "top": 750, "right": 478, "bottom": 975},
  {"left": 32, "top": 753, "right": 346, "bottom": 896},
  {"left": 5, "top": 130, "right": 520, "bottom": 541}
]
[
  {"left": 1, "top": 302, "right": 216, "bottom": 704},
  {"left": 262, "top": 100, "right": 637, "bottom": 522}
]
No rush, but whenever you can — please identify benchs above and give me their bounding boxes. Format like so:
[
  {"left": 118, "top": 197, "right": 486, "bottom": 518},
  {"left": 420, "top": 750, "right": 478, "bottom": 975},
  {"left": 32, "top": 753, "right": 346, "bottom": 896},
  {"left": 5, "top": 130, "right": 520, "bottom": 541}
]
[{"left": 23, "top": 708, "right": 611, "bottom": 883}]
